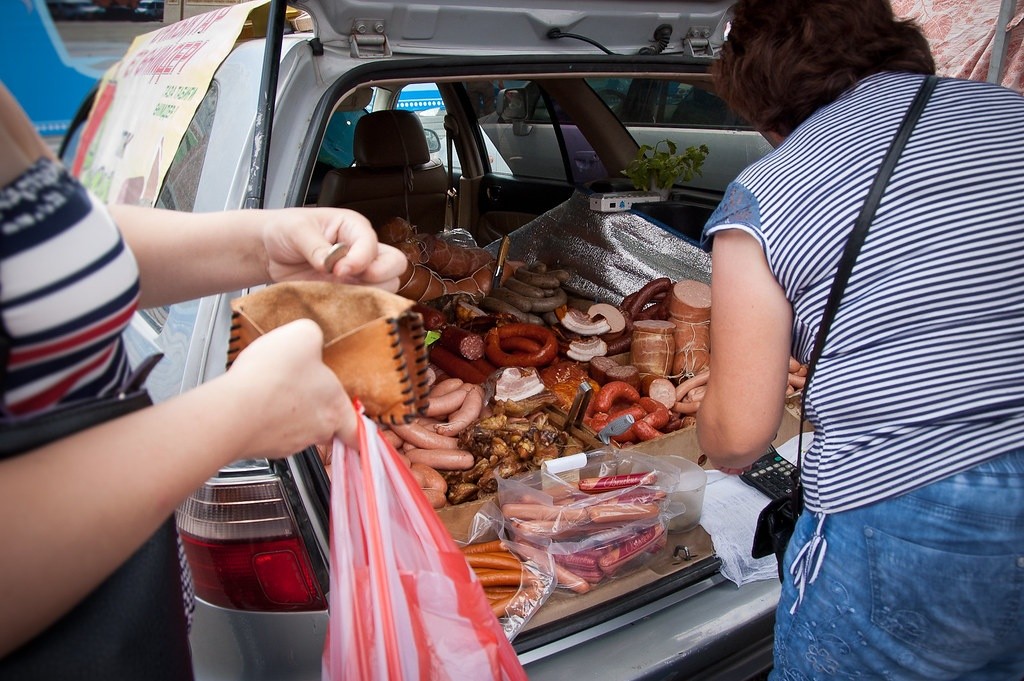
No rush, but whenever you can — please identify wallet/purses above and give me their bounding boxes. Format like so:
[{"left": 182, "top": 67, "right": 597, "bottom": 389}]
[{"left": 226, "top": 279, "right": 431, "bottom": 426}]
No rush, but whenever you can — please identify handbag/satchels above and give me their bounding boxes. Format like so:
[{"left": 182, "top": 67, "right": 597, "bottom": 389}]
[
  {"left": 750, "top": 493, "right": 805, "bottom": 585},
  {"left": 0, "top": 282, "right": 198, "bottom": 681}
]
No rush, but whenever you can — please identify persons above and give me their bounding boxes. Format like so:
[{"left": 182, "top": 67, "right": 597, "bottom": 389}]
[
  {"left": 695, "top": 0, "right": 1024, "bottom": 677},
  {"left": 1, "top": 77, "right": 411, "bottom": 681}
]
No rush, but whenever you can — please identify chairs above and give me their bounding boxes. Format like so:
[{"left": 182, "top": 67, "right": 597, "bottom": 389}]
[{"left": 315, "top": 107, "right": 449, "bottom": 237}]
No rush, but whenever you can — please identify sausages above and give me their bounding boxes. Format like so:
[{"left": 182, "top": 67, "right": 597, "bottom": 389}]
[{"left": 312, "top": 216, "right": 808, "bottom": 627}]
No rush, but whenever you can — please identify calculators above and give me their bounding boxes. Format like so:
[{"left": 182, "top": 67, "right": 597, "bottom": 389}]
[{"left": 736, "top": 444, "right": 803, "bottom": 499}]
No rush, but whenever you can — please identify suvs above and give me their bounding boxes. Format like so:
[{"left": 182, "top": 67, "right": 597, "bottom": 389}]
[
  {"left": 74, "top": 0, "right": 813, "bottom": 681},
  {"left": 405, "top": 61, "right": 789, "bottom": 198}
]
